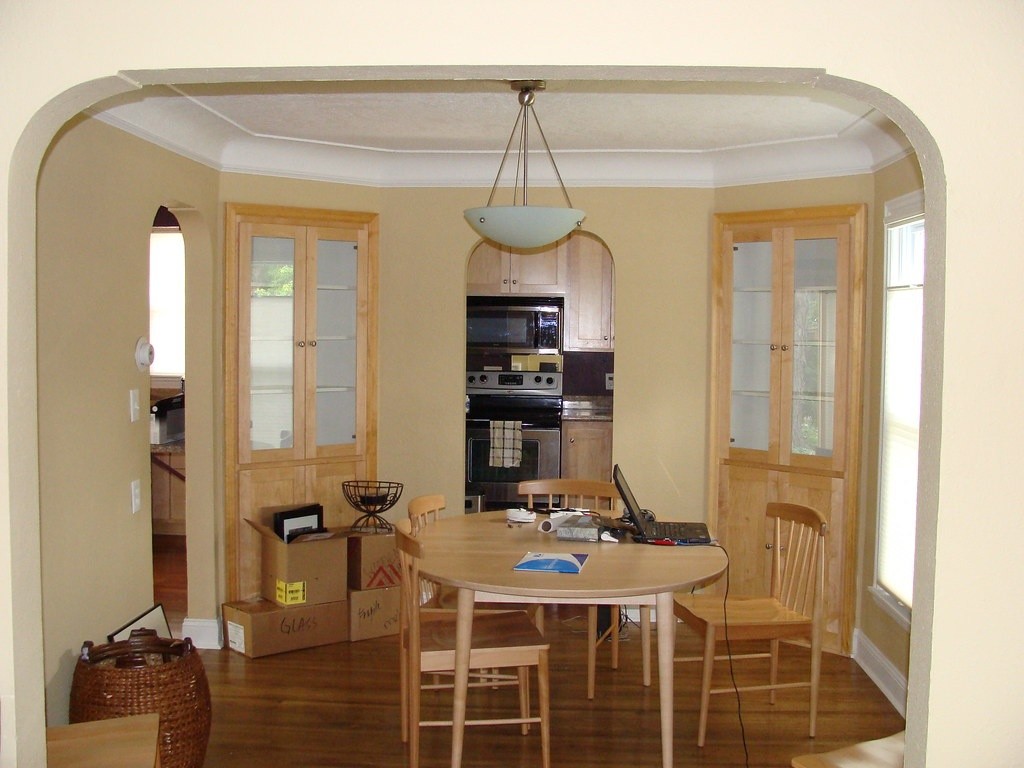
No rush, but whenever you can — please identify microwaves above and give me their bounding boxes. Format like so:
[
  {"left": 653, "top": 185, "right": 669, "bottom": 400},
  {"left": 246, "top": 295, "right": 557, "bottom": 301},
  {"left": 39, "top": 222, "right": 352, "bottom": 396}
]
[{"left": 468, "top": 296, "right": 563, "bottom": 354}]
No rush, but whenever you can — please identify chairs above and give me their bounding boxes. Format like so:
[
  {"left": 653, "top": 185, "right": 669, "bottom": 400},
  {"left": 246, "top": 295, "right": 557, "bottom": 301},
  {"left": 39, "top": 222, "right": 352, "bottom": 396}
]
[
  {"left": 408, "top": 494, "right": 531, "bottom": 731},
  {"left": 518, "top": 479, "right": 623, "bottom": 699},
  {"left": 394, "top": 526, "right": 550, "bottom": 768},
  {"left": 672, "top": 502, "right": 826, "bottom": 748}
]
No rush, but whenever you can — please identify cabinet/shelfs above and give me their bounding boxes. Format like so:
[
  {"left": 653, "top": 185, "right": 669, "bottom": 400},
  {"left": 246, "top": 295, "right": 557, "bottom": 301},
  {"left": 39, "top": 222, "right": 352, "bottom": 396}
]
[
  {"left": 467, "top": 231, "right": 614, "bottom": 352},
  {"left": 560, "top": 421, "right": 613, "bottom": 511}
]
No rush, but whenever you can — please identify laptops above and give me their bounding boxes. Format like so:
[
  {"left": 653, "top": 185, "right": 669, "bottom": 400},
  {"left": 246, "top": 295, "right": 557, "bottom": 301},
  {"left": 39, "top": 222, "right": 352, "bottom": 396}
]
[{"left": 613, "top": 464, "right": 711, "bottom": 543}]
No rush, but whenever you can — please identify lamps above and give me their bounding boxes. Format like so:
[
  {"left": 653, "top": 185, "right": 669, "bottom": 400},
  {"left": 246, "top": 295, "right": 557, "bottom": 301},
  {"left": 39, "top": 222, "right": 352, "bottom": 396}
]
[{"left": 464, "top": 80, "right": 586, "bottom": 248}]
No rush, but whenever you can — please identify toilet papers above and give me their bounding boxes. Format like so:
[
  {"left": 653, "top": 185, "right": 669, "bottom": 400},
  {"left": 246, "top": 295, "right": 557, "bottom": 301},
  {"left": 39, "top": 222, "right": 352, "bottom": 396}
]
[{"left": 537, "top": 512, "right": 574, "bottom": 533}]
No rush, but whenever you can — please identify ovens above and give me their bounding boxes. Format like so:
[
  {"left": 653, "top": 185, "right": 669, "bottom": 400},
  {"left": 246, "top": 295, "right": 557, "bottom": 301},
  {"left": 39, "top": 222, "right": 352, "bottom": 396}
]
[{"left": 466, "top": 354, "right": 562, "bottom": 505}]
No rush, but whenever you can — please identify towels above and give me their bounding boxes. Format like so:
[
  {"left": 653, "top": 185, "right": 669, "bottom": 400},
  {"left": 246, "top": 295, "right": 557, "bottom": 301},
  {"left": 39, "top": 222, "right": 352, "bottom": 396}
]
[{"left": 488, "top": 420, "right": 522, "bottom": 467}]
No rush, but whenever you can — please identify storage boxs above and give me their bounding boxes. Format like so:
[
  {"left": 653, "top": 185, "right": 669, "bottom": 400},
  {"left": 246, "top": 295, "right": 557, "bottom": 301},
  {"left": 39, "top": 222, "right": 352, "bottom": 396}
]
[{"left": 222, "top": 516, "right": 401, "bottom": 659}]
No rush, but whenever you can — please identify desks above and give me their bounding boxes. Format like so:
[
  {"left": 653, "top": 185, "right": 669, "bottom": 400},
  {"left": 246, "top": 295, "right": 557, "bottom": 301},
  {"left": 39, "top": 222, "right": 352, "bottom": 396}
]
[
  {"left": 404, "top": 509, "right": 728, "bottom": 768},
  {"left": 46, "top": 713, "right": 162, "bottom": 768}
]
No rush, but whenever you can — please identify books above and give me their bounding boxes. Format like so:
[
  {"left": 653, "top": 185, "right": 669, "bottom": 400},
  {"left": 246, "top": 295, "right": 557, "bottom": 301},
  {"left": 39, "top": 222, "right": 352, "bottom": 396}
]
[{"left": 513, "top": 551, "right": 589, "bottom": 574}]
